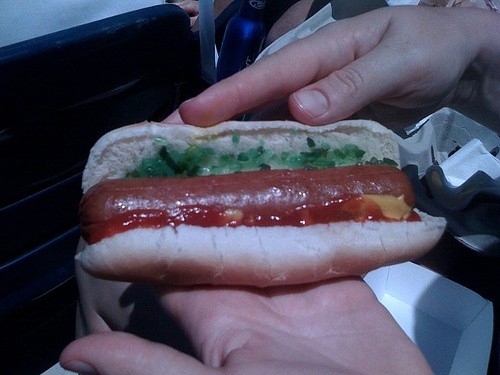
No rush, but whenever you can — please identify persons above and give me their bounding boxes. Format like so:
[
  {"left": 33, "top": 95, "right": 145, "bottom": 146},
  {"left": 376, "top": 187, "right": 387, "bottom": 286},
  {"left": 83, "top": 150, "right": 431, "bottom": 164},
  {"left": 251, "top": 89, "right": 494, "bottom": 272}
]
[{"left": 59, "top": 5, "right": 500, "bottom": 373}]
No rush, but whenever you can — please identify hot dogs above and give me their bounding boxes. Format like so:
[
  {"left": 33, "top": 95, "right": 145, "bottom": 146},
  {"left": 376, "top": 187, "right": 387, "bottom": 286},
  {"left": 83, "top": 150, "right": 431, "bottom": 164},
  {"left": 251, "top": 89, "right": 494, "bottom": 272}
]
[{"left": 74, "top": 120, "right": 446, "bottom": 289}]
